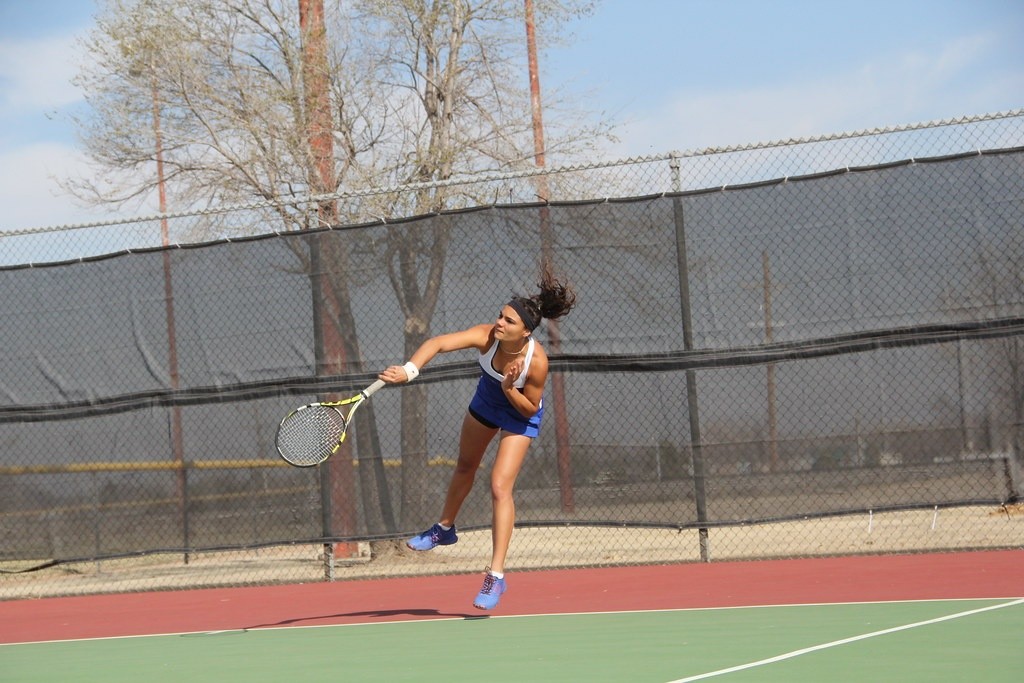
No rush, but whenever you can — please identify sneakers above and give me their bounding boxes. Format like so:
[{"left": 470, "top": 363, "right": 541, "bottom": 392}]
[
  {"left": 406, "top": 523, "right": 458, "bottom": 551},
  {"left": 473, "top": 575, "right": 506, "bottom": 610}
]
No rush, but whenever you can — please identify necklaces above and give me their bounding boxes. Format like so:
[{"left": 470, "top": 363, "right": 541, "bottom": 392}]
[{"left": 498, "top": 338, "right": 528, "bottom": 355}]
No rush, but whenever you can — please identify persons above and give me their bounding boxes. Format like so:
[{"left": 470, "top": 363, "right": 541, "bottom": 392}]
[{"left": 378, "top": 256, "right": 577, "bottom": 609}]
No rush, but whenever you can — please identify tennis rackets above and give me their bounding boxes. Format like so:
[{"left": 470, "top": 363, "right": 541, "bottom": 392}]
[{"left": 274, "top": 379, "right": 387, "bottom": 469}]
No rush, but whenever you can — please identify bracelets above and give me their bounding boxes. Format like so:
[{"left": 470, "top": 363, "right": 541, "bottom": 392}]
[{"left": 402, "top": 361, "right": 420, "bottom": 383}]
